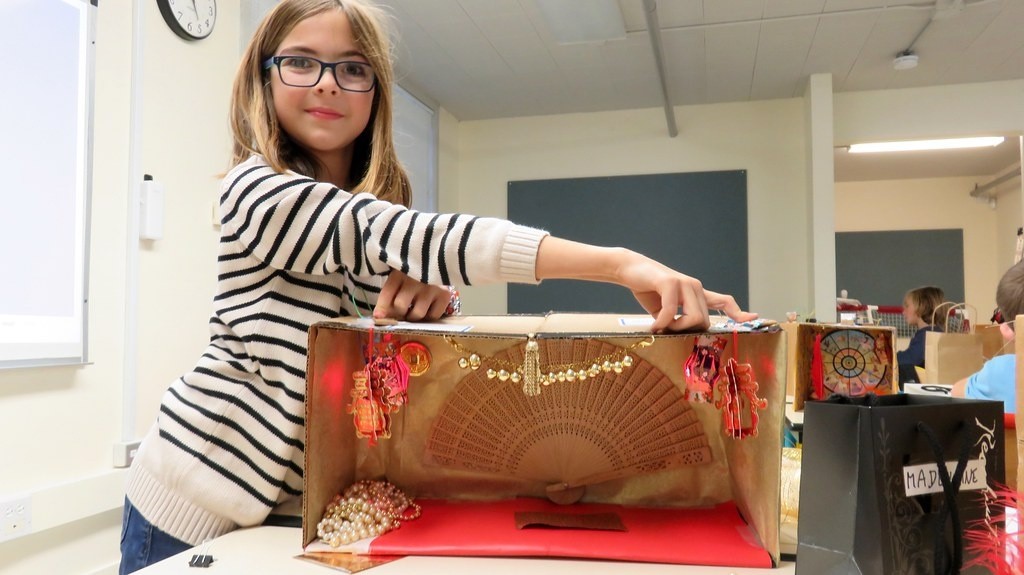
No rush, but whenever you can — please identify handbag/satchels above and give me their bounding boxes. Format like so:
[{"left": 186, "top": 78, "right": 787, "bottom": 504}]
[
  {"left": 924, "top": 302, "right": 983, "bottom": 385},
  {"left": 796, "top": 392, "right": 1005, "bottom": 575}
]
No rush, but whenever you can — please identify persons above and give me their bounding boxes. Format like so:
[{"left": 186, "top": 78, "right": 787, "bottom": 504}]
[
  {"left": 949, "top": 259, "right": 1023, "bottom": 414},
  {"left": 118, "top": 0, "right": 760, "bottom": 574},
  {"left": 896, "top": 285, "right": 946, "bottom": 392}
]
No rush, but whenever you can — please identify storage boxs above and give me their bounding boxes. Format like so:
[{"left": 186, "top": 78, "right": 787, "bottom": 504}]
[
  {"left": 304, "top": 314, "right": 788, "bottom": 568},
  {"left": 793, "top": 325, "right": 900, "bottom": 413}
]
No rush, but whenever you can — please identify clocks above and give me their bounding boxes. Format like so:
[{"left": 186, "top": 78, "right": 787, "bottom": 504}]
[{"left": 156, "top": 0, "right": 217, "bottom": 41}]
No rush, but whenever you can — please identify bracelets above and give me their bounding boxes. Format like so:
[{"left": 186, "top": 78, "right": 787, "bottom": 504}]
[{"left": 443, "top": 284, "right": 461, "bottom": 315}]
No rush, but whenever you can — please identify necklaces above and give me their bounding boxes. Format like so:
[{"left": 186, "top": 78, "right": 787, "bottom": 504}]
[{"left": 315, "top": 478, "right": 423, "bottom": 548}]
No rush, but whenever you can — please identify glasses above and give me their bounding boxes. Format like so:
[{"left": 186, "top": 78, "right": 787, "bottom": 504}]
[{"left": 264, "top": 55, "right": 378, "bottom": 91}]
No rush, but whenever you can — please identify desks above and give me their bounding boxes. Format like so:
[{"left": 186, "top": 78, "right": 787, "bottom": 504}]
[{"left": 129, "top": 526, "right": 796, "bottom": 575}]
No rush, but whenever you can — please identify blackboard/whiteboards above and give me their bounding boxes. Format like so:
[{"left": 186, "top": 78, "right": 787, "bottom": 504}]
[
  {"left": 389, "top": 76, "right": 443, "bottom": 213},
  {"left": 0, "top": 0, "right": 100, "bottom": 375}
]
